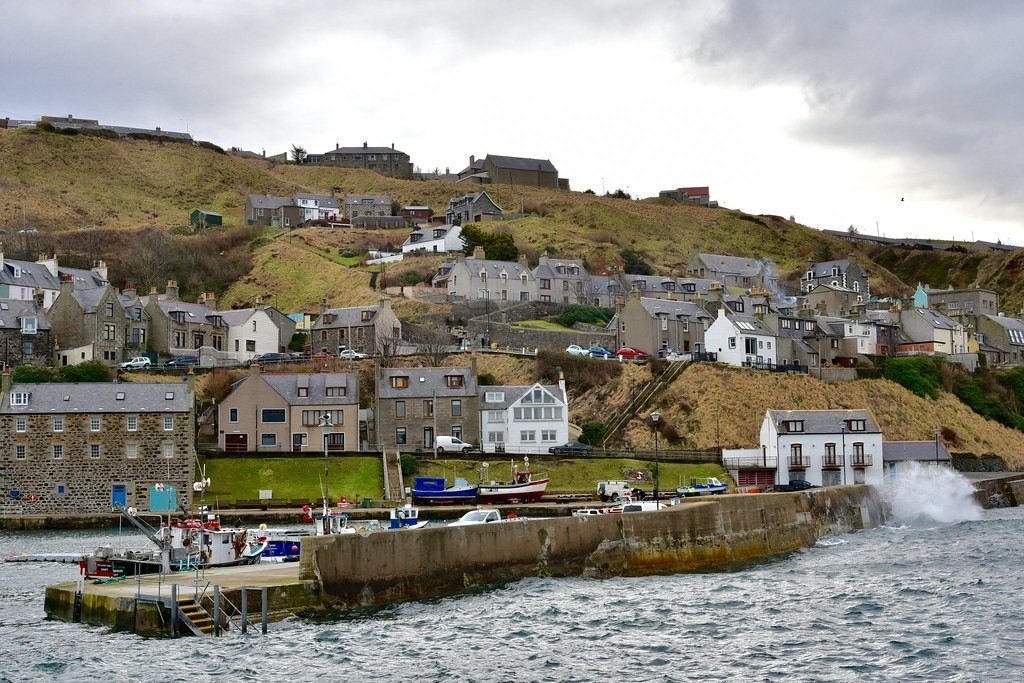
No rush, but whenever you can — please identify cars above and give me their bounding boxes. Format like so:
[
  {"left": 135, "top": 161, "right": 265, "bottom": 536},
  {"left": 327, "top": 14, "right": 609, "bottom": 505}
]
[
  {"left": 566, "top": 344, "right": 589, "bottom": 357},
  {"left": 621, "top": 501, "right": 668, "bottom": 514},
  {"left": 548, "top": 441, "right": 594, "bottom": 457},
  {"left": 164, "top": 354, "right": 198, "bottom": 368},
  {"left": 571, "top": 506, "right": 622, "bottom": 517},
  {"left": 588, "top": 346, "right": 615, "bottom": 359},
  {"left": 616, "top": 347, "right": 648, "bottom": 361},
  {"left": 251, "top": 348, "right": 369, "bottom": 366}
]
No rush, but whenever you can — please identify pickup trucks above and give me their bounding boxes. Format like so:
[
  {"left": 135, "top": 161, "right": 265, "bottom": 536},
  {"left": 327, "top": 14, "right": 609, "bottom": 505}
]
[
  {"left": 774, "top": 479, "right": 812, "bottom": 492},
  {"left": 447, "top": 509, "right": 527, "bottom": 527}
]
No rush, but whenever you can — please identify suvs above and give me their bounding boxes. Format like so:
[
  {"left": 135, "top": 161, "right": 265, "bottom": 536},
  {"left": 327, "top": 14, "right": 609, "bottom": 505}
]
[
  {"left": 596, "top": 480, "right": 636, "bottom": 502},
  {"left": 116, "top": 356, "right": 151, "bottom": 371}
]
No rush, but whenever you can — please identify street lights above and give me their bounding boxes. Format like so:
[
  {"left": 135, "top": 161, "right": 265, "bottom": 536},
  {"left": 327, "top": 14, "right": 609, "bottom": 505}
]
[
  {"left": 838, "top": 421, "right": 848, "bottom": 486},
  {"left": 650, "top": 411, "right": 661, "bottom": 501},
  {"left": 199, "top": 323, "right": 204, "bottom": 365},
  {"left": 318, "top": 414, "right": 335, "bottom": 536},
  {"left": 932, "top": 424, "right": 941, "bottom": 472}
]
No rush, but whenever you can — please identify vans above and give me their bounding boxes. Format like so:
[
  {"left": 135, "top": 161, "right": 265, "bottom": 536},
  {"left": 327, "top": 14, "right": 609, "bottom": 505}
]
[{"left": 433, "top": 435, "right": 476, "bottom": 454}]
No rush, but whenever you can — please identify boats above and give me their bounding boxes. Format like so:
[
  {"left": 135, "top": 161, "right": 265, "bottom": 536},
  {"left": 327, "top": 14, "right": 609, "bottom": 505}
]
[
  {"left": 675, "top": 476, "right": 728, "bottom": 498},
  {"left": 79, "top": 448, "right": 352, "bottom": 579},
  {"left": 412, "top": 476, "right": 479, "bottom": 505},
  {"left": 387, "top": 504, "right": 430, "bottom": 529},
  {"left": 479, "top": 454, "right": 550, "bottom": 502}
]
